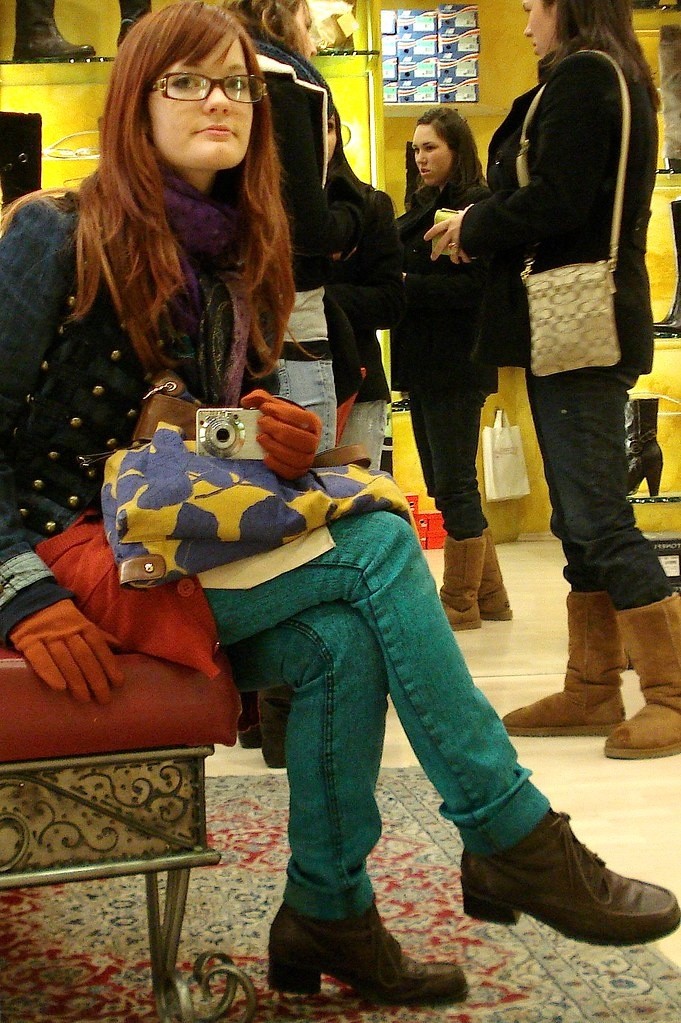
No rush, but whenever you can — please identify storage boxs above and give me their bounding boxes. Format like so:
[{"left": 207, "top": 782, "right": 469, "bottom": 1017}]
[
  {"left": 405, "top": 494, "right": 448, "bottom": 550},
  {"left": 380, "top": 4, "right": 481, "bottom": 103}
]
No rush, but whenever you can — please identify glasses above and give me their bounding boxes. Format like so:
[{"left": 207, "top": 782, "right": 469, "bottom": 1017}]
[{"left": 145, "top": 71, "right": 269, "bottom": 104}]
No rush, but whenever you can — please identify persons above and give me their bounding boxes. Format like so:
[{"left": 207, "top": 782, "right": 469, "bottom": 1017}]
[
  {"left": 234, "top": 0, "right": 681, "bottom": 761},
  {"left": 0, "top": 0, "right": 681, "bottom": 1000}
]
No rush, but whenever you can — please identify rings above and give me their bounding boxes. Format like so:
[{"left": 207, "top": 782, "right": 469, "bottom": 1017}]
[{"left": 448, "top": 242, "right": 459, "bottom": 248}]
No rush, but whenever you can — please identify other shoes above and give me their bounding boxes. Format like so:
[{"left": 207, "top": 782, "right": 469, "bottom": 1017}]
[
  {"left": 240, "top": 728, "right": 262, "bottom": 748},
  {"left": 262, "top": 739, "right": 286, "bottom": 768}
]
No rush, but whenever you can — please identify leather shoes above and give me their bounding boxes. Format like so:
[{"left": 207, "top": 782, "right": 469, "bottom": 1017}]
[
  {"left": 267, "top": 901, "right": 469, "bottom": 1007},
  {"left": 461, "top": 807, "right": 681, "bottom": 946}
]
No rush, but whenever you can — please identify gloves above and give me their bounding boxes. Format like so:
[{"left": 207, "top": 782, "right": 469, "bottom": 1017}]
[
  {"left": 240, "top": 388, "right": 322, "bottom": 479},
  {"left": 9, "top": 597, "right": 126, "bottom": 705}
]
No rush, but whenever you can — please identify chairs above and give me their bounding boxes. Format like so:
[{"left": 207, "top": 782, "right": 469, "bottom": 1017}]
[{"left": 0, "top": 642, "right": 257, "bottom": 1023}]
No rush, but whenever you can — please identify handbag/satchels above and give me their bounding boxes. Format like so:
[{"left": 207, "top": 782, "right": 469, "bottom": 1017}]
[
  {"left": 482, "top": 409, "right": 530, "bottom": 502},
  {"left": 103, "top": 371, "right": 418, "bottom": 589},
  {"left": 522, "top": 261, "right": 624, "bottom": 375}
]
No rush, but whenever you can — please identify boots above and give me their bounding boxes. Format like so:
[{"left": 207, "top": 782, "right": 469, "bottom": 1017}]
[
  {"left": 435, "top": 536, "right": 488, "bottom": 631},
  {"left": 619, "top": 400, "right": 665, "bottom": 497},
  {"left": 604, "top": 593, "right": 681, "bottom": 759},
  {"left": 9, "top": 1, "right": 95, "bottom": 59},
  {"left": 478, "top": 533, "right": 513, "bottom": 621},
  {"left": 503, "top": 593, "right": 628, "bottom": 737},
  {"left": 653, "top": 201, "right": 681, "bottom": 340}
]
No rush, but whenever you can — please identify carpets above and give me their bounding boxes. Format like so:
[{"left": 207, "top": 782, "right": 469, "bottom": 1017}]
[{"left": 0, "top": 764, "right": 681, "bottom": 1023}]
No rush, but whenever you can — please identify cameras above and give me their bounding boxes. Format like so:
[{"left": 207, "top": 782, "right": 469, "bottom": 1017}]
[{"left": 195, "top": 406, "right": 268, "bottom": 460}]
